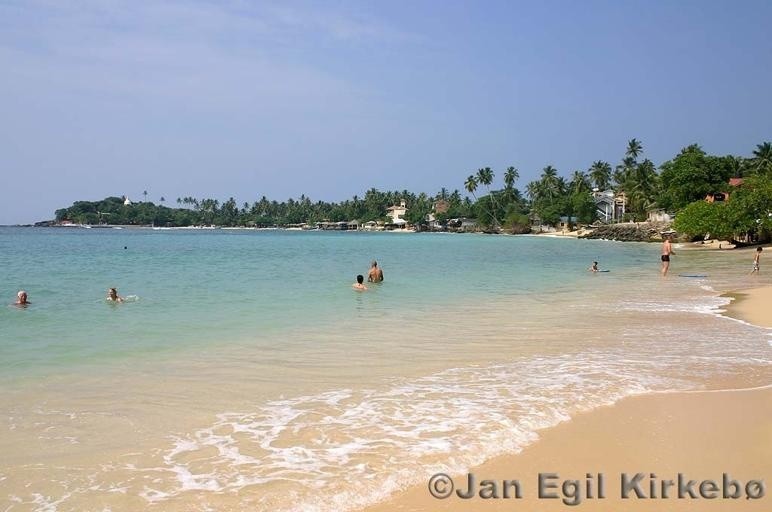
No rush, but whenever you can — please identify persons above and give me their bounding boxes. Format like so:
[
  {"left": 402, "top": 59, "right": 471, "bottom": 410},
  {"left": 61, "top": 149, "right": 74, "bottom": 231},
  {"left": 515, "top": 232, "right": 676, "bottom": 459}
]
[
  {"left": 588, "top": 262, "right": 599, "bottom": 272},
  {"left": 106, "top": 288, "right": 121, "bottom": 302},
  {"left": 660, "top": 236, "right": 676, "bottom": 276},
  {"left": 752, "top": 247, "right": 763, "bottom": 274},
  {"left": 368, "top": 259, "right": 384, "bottom": 283},
  {"left": 10, "top": 290, "right": 31, "bottom": 308},
  {"left": 352, "top": 274, "right": 368, "bottom": 290}
]
